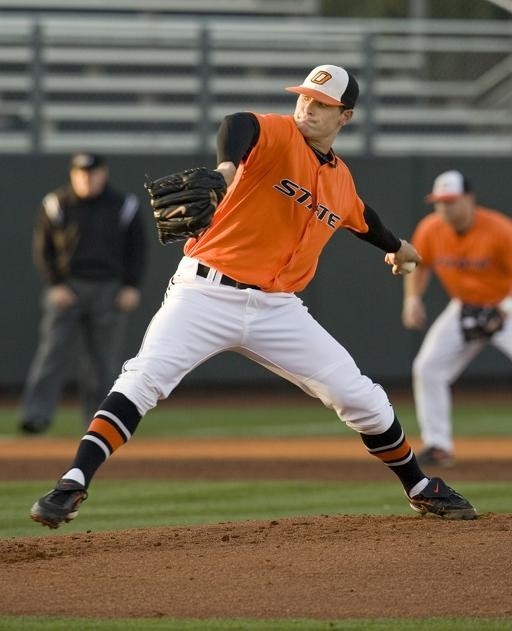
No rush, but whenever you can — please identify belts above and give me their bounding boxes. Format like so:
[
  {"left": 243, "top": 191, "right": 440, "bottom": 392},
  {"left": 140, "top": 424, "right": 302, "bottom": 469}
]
[{"left": 197, "top": 263, "right": 261, "bottom": 289}]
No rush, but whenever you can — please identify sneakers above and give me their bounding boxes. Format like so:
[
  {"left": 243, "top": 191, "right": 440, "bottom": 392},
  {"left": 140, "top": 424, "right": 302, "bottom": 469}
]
[
  {"left": 404, "top": 476, "right": 476, "bottom": 517},
  {"left": 31, "top": 464, "right": 89, "bottom": 528}
]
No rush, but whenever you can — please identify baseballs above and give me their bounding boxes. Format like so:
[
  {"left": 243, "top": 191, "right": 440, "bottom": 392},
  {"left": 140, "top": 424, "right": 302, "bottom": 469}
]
[{"left": 399, "top": 260, "right": 415, "bottom": 274}]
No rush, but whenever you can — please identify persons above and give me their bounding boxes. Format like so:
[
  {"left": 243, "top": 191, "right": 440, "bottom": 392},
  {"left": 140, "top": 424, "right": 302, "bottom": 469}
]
[
  {"left": 400, "top": 170, "right": 511, "bottom": 471},
  {"left": 28, "top": 64, "right": 478, "bottom": 532},
  {"left": 17, "top": 152, "right": 151, "bottom": 435}
]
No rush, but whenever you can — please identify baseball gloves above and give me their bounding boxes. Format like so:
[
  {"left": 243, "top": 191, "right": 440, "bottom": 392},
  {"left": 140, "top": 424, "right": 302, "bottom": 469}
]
[
  {"left": 460, "top": 302, "right": 504, "bottom": 341},
  {"left": 144, "top": 168, "right": 226, "bottom": 245}
]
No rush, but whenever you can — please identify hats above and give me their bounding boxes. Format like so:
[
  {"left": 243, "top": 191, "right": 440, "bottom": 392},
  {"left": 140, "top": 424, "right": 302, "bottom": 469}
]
[
  {"left": 426, "top": 170, "right": 474, "bottom": 201},
  {"left": 287, "top": 64, "right": 358, "bottom": 106}
]
